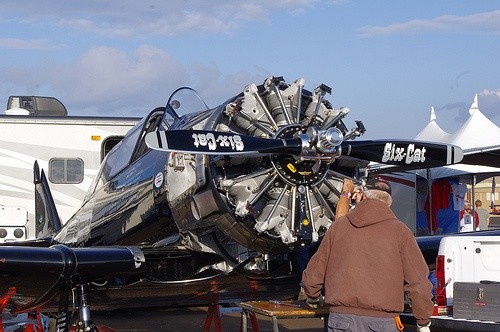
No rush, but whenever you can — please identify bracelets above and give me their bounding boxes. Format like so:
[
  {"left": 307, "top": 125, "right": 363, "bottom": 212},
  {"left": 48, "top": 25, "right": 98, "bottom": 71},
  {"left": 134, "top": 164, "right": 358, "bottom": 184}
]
[{"left": 342, "top": 190, "right": 352, "bottom": 199}]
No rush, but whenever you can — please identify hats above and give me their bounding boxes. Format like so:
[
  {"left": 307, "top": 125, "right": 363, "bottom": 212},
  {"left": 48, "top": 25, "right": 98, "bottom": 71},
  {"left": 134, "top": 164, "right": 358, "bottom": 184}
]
[{"left": 362, "top": 175, "right": 391, "bottom": 196}]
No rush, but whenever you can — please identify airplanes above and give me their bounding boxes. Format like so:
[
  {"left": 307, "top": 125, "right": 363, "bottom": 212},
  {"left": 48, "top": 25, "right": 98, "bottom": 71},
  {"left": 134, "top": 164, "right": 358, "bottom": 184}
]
[{"left": 0, "top": 78, "right": 499, "bottom": 332}]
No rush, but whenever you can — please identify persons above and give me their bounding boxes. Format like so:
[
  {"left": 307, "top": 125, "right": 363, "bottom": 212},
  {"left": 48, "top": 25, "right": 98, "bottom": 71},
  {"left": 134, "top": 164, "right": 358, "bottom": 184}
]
[
  {"left": 335, "top": 178, "right": 362, "bottom": 223},
  {"left": 302, "top": 180, "right": 433, "bottom": 332},
  {"left": 459, "top": 199, "right": 490, "bottom": 233}
]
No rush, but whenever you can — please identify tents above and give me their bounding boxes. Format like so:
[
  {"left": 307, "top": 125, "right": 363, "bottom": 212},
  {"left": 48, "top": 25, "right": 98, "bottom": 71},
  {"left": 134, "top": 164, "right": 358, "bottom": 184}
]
[{"left": 413, "top": 93, "right": 500, "bottom": 236}]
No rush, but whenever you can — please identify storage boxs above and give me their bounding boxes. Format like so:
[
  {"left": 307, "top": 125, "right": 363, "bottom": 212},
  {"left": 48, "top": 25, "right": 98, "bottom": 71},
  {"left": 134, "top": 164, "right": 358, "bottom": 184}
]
[{"left": 452, "top": 282, "right": 500, "bottom": 322}]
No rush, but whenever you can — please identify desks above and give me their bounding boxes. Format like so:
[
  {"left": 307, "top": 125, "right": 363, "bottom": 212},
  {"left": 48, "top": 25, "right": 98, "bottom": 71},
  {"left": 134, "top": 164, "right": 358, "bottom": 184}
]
[
  {"left": 399, "top": 311, "right": 500, "bottom": 332},
  {"left": 241, "top": 301, "right": 330, "bottom": 332}
]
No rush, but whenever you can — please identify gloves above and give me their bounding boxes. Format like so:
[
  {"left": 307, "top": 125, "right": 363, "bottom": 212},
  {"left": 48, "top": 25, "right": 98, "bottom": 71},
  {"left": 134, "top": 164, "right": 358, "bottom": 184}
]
[
  {"left": 306, "top": 293, "right": 324, "bottom": 308},
  {"left": 417, "top": 321, "right": 432, "bottom": 332}
]
